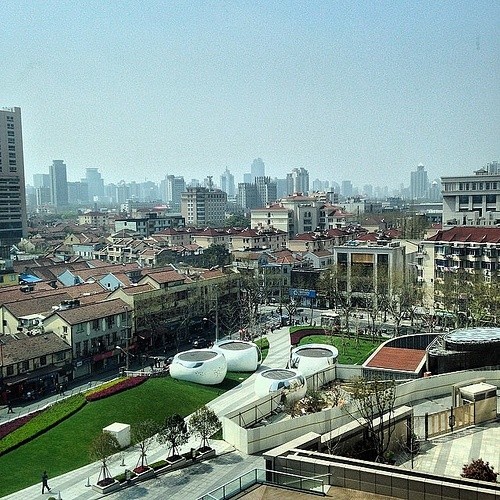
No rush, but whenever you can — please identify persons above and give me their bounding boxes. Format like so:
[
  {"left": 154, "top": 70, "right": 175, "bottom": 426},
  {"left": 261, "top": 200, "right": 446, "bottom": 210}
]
[
  {"left": 42, "top": 471, "right": 51, "bottom": 494},
  {"left": 7, "top": 379, "right": 69, "bottom": 414},
  {"left": 271, "top": 306, "right": 366, "bottom": 326},
  {"left": 137, "top": 334, "right": 194, "bottom": 369}
]
